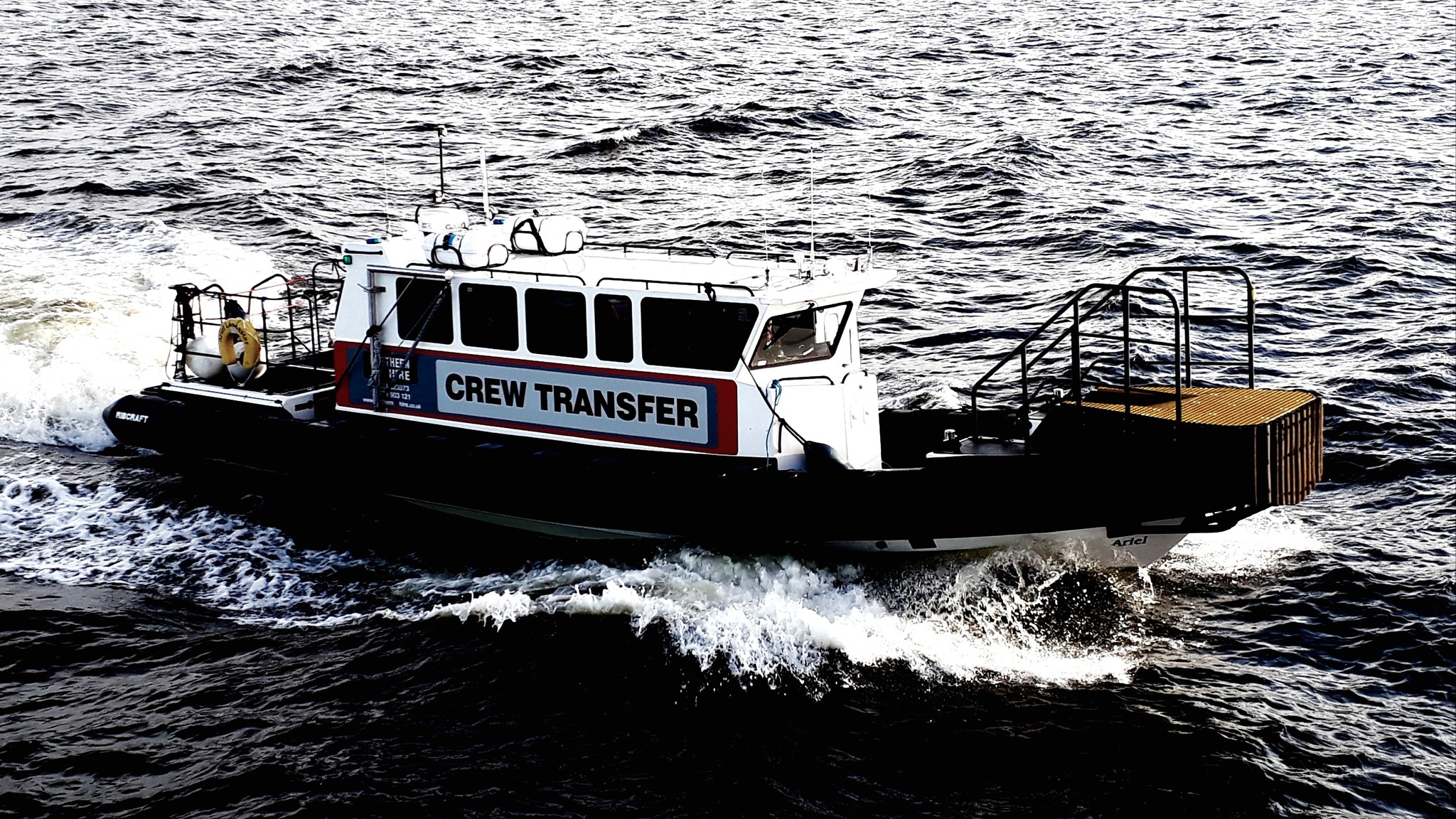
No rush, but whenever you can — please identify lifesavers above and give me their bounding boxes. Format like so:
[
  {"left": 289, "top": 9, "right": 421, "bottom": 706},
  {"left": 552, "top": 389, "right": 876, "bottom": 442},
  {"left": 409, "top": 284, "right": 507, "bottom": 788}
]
[{"left": 218, "top": 318, "right": 262, "bottom": 369}]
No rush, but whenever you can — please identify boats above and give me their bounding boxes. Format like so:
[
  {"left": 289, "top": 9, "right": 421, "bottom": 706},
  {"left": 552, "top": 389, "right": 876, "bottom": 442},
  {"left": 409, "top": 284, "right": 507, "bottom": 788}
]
[{"left": 100, "top": 124, "right": 1326, "bottom": 579}]
